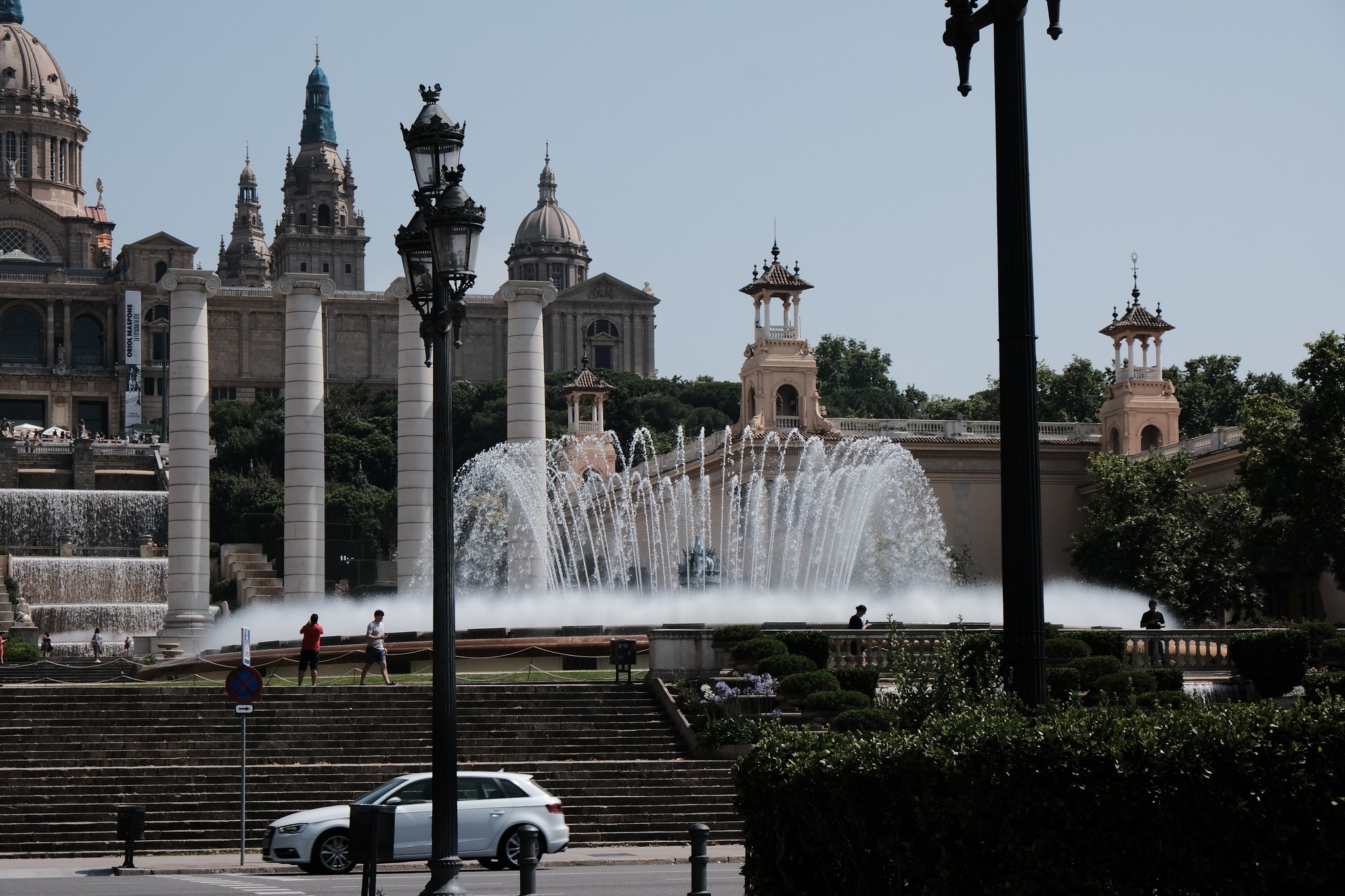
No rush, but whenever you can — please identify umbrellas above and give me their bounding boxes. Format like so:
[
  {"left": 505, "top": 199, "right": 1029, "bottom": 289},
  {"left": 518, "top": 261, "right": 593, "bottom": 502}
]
[
  {"left": 7, "top": 423, "right": 66, "bottom": 438},
  {"left": 145, "top": 433, "right": 153, "bottom": 436}
]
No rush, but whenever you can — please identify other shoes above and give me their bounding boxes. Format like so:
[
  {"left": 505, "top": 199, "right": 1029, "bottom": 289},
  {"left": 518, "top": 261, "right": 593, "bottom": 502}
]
[
  {"left": 94, "top": 659, "right": 102, "bottom": 663},
  {"left": 387, "top": 681, "right": 399, "bottom": 685}
]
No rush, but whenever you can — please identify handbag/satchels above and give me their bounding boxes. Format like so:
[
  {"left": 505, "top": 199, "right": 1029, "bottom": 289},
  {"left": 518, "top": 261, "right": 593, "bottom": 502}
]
[{"left": 90, "top": 642, "right": 94, "bottom": 647}]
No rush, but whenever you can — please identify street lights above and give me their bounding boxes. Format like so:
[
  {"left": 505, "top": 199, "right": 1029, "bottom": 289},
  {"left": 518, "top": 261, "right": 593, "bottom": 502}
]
[{"left": 392, "top": 85, "right": 487, "bottom": 896}]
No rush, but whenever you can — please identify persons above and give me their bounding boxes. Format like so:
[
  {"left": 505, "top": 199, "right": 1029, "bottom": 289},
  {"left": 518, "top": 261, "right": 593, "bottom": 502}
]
[
  {"left": 78, "top": 419, "right": 85, "bottom": 438},
  {"left": 0, "top": 418, "right": 15, "bottom": 437},
  {"left": 1140, "top": 599, "right": 1166, "bottom": 666},
  {"left": 298, "top": 613, "right": 323, "bottom": 686},
  {"left": 848, "top": 605, "right": 872, "bottom": 666},
  {"left": 161, "top": 456, "right": 169, "bottom": 465},
  {"left": 81, "top": 628, "right": 103, "bottom": 663},
  {"left": 15, "top": 598, "right": 31, "bottom": 622},
  {"left": 124, "top": 636, "right": 131, "bottom": 657},
  {"left": 42, "top": 632, "right": 51, "bottom": 657},
  {"left": 359, "top": 610, "right": 394, "bottom": 686},
  {"left": 0, "top": 631, "right": 7, "bottom": 664},
  {"left": 20, "top": 428, "right": 150, "bottom": 452}
]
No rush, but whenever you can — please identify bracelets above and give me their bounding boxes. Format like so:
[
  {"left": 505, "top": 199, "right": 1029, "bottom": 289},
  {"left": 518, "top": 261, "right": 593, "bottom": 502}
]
[{"left": 383, "top": 647, "right": 386, "bottom": 648}]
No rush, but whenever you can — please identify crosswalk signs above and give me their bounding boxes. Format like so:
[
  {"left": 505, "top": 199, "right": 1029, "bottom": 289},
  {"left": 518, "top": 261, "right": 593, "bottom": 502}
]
[{"left": 241, "top": 627, "right": 251, "bottom": 666}]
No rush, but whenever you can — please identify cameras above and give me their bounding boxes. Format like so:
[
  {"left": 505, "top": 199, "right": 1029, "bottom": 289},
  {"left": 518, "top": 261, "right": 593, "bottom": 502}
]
[{"left": 1153, "top": 619, "right": 1160, "bottom": 625}]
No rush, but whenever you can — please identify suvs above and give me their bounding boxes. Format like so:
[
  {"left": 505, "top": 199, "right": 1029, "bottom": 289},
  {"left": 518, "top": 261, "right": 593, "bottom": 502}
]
[{"left": 260, "top": 768, "right": 570, "bottom": 876}]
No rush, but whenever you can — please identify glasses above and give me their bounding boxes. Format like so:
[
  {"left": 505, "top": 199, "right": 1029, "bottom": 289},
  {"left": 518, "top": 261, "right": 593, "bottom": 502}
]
[{"left": 99, "top": 629, "right": 101, "bottom": 630}]
